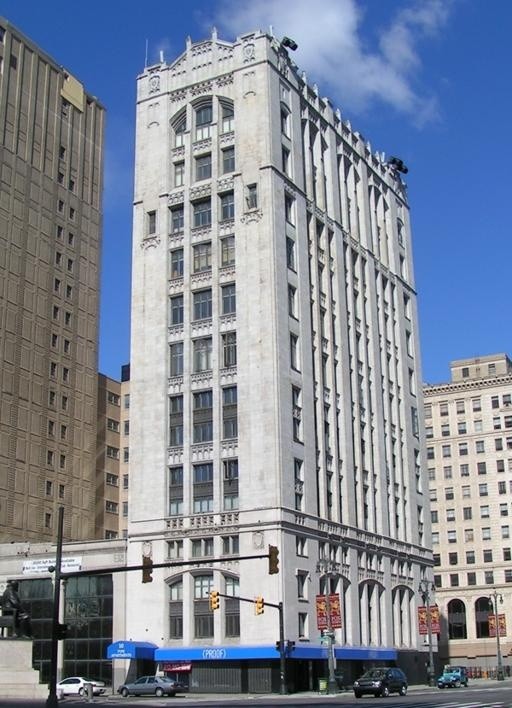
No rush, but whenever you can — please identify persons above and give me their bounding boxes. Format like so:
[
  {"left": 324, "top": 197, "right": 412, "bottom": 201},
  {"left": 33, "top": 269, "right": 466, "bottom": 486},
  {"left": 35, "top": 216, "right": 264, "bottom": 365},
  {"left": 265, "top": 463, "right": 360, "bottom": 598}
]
[{"left": 3, "top": 579, "right": 35, "bottom": 640}]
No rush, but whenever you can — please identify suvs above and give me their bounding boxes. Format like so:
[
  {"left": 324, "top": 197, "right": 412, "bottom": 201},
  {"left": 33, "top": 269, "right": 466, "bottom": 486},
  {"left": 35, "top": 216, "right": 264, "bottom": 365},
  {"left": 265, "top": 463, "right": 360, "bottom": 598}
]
[{"left": 438, "top": 665, "right": 468, "bottom": 688}]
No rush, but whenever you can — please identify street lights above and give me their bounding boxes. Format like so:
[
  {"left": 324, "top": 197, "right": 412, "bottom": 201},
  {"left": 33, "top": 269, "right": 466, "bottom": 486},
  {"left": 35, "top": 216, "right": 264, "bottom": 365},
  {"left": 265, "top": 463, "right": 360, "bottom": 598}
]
[
  {"left": 315, "top": 557, "right": 340, "bottom": 694},
  {"left": 418, "top": 578, "right": 436, "bottom": 687},
  {"left": 488, "top": 590, "right": 505, "bottom": 680}
]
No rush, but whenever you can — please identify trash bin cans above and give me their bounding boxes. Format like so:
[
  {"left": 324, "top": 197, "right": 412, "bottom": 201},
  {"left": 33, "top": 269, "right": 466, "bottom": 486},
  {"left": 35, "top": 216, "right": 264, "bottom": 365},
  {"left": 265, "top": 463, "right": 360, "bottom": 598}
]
[
  {"left": 497, "top": 671, "right": 504, "bottom": 681},
  {"left": 83, "top": 683, "right": 93, "bottom": 700}
]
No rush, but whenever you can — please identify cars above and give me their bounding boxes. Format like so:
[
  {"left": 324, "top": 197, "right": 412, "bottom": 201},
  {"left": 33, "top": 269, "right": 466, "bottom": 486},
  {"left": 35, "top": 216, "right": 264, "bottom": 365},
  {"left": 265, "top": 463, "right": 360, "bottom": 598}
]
[
  {"left": 117, "top": 676, "right": 186, "bottom": 697},
  {"left": 353, "top": 667, "right": 408, "bottom": 698},
  {"left": 56, "top": 677, "right": 106, "bottom": 696}
]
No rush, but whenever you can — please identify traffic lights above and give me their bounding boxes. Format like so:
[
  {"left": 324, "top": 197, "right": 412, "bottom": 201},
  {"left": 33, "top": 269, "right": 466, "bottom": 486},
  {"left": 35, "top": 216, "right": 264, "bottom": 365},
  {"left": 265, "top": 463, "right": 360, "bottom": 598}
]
[
  {"left": 268, "top": 544, "right": 280, "bottom": 575},
  {"left": 256, "top": 596, "right": 264, "bottom": 616},
  {"left": 209, "top": 591, "right": 220, "bottom": 611},
  {"left": 57, "top": 622, "right": 68, "bottom": 641},
  {"left": 142, "top": 556, "right": 153, "bottom": 584},
  {"left": 276, "top": 639, "right": 296, "bottom": 653}
]
